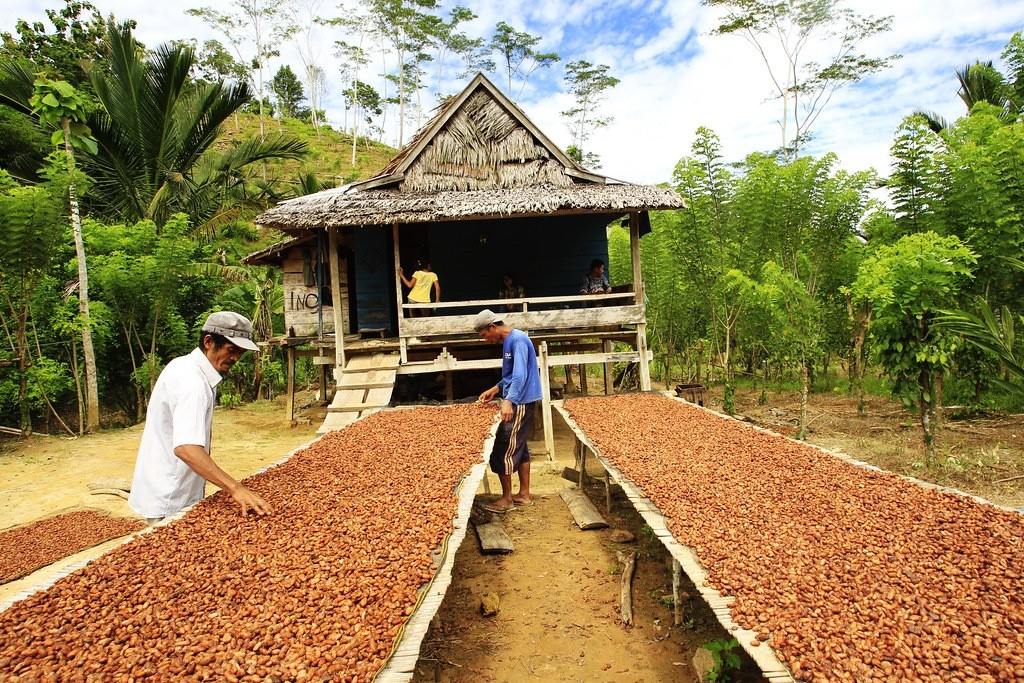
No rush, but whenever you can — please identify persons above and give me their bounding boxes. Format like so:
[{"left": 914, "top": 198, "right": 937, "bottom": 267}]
[
  {"left": 398, "top": 256, "right": 440, "bottom": 318},
  {"left": 498, "top": 274, "right": 526, "bottom": 313},
  {"left": 473, "top": 309, "right": 543, "bottom": 514},
  {"left": 538, "top": 270, "right": 577, "bottom": 309},
  {"left": 127, "top": 311, "right": 273, "bottom": 526},
  {"left": 579, "top": 258, "right": 612, "bottom": 307}
]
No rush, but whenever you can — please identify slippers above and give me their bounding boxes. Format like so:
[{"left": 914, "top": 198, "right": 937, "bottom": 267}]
[
  {"left": 484, "top": 503, "right": 516, "bottom": 513},
  {"left": 512, "top": 498, "right": 533, "bottom": 506}
]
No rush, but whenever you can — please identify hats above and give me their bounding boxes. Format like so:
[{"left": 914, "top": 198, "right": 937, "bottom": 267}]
[
  {"left": 474, "top": 309, "right": 507, "bottom": 331},
  {"left": 202, "top": 312, "right": 260, "bottom": 351}
]
